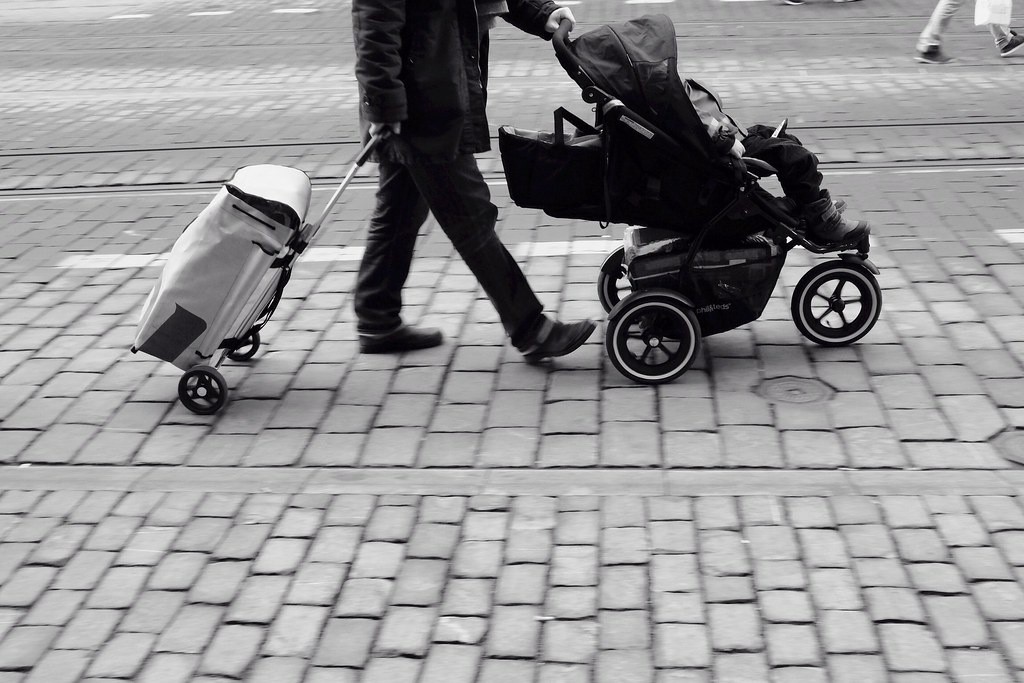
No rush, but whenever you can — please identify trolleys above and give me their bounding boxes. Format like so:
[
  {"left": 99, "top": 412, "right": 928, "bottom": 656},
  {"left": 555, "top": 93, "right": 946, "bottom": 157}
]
[{"left": 177, "top": 123, "right": 394, "bottom": 416}]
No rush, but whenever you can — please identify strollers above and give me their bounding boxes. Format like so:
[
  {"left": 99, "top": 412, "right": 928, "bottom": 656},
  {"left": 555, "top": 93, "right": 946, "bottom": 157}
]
[{"left": 497, "top": 14, "right": 883, "bottom": 384}]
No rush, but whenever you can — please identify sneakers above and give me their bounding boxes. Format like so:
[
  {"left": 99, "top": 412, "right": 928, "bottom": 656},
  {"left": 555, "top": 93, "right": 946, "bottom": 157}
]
[
  {"left": 785, "top": 0, "right": 805, "bottom": 5},
  {"left": 832, "top": 198, "right": 848, "bottom": 215},
  {"left": 791, "top": 188, "right": 872, "bottom": 249},
  {"left": 913, "top": 44, "right": 958, "bottom": 65},
  {"left": 833, "top": 0, "right": 859, "bottom": 3},
  {"left": 998, "top": 34, "right": 1024, "bottom": 57}
]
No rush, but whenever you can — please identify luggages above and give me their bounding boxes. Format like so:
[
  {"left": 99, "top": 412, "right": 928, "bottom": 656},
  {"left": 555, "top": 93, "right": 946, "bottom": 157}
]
[{"left": 131, "top": 128, "right": 388, "bottom": 416}]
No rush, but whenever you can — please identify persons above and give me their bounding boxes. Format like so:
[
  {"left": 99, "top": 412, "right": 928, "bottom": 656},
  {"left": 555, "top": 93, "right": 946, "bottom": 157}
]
[
  {"left": 684, "top": 76, "right": 870, "bottom": 247},
  {"left": 352, "top": 0, "right": 599, "bottom": 364},
  {"left": 914, "top": 0, "right": 1024, "bottom": 63}
]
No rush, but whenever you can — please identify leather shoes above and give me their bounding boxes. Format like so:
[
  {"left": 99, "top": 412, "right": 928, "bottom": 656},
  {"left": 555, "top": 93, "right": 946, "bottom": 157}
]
[
  {"left": 511, "top": 313, "right": 598, "bottom": 365},
  {"left": 358, "top": 322, "right": 442, "bottom": 355}
]
